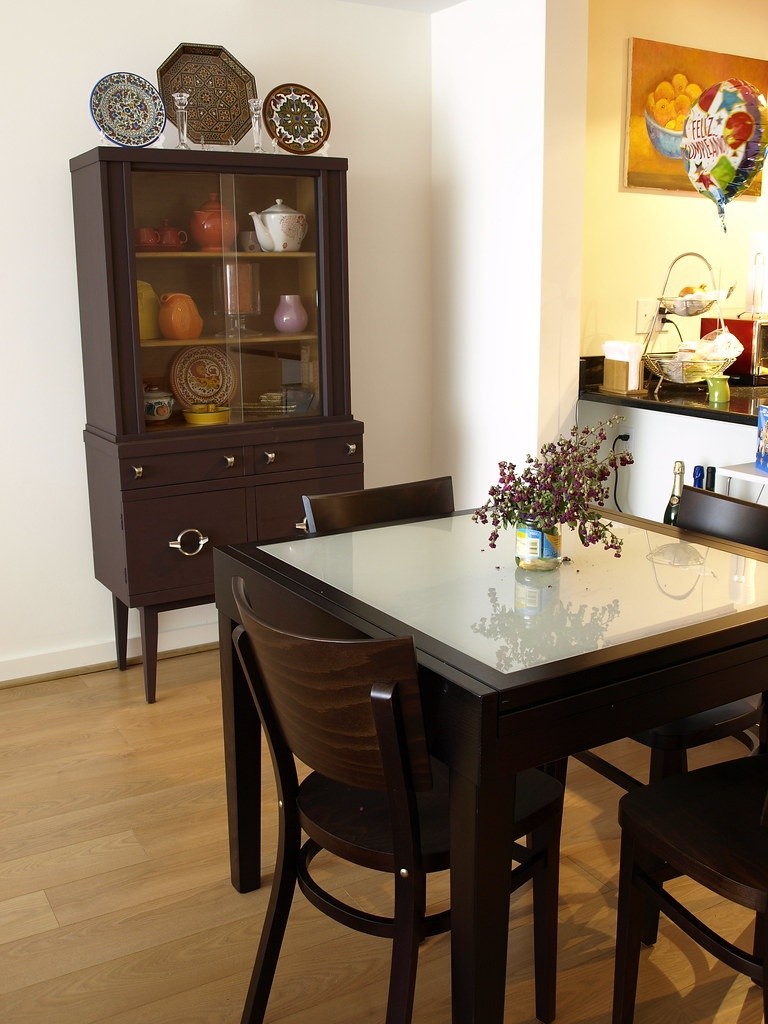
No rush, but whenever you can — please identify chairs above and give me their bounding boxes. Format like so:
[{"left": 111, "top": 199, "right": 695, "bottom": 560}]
[
  {"left": 536, "top": 485, "right": 767, "bottom": 947},
  {"left": 301, "top": 476, "right": 456, "bottom": 533},
  {"left": 612, "top": 755, "right": 768, "bottom": 1023},
  {"left": 231, "top": 576, "right": 564, "bottom": 1024}
]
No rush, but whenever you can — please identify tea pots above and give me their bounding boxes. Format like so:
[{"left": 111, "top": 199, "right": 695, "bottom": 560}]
[{"left": 248, "top": 198, "right": 308, "bottom": 252}]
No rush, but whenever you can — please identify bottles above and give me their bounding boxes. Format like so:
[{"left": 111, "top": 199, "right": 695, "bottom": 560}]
[
  {"left": 663, "top": 461, "right": 716, "bottom": 528},
  {"left": 274, "top": 295, "right": 308, "bottom": 334}
]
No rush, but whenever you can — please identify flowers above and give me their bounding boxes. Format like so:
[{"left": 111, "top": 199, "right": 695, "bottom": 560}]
[{"left": 470, "top": 414, "right": 634, "bottom": 558}]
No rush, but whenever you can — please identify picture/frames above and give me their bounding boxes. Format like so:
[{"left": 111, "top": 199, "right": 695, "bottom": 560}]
[{"left": 617, "top": 36, "right": 768, "bottom": 204}]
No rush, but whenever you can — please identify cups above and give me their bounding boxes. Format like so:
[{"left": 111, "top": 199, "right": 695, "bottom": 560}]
[
  {"left": 133, "top": 227, "right": 160, "bottom": 251},
  {"left": 160, "top": 226, "right": 188, "bottom": 251},
  {"left": 238, "top": 231, "right": 261, "bottom": 253}
]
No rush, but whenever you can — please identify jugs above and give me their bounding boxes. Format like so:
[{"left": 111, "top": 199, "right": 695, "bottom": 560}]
[
  {"left": 701, "top": 375, "right": 731, "bottom": 403},
  {"left": 160, "top": 292, "right": 204, "bottom": 340},
  {"left": 191, "top": 191, "right": 239, "bottom": 252},
  {"left": 136, "top": 281, "right": 161, "bottom": 340}
]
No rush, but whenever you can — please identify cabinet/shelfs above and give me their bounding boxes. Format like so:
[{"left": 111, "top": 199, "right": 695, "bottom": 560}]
[{"left": 69, "top": 146, "right": 366, "bottom": 705}]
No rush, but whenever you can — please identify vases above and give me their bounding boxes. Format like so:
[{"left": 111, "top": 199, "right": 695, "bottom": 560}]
[{"left": 514, "top": 514, "right": 562, "bottom": 570}]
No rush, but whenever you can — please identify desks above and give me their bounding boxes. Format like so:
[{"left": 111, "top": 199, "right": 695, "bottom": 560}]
[
  {"left": 212, "top": 503, "right": 768, "bottom": 1024},
  {"left": 716, "top": 462, "right": 768, "bottom": 505}
]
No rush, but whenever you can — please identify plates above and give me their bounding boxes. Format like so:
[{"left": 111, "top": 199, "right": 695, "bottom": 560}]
[
  {"left": 169, "top": 346, "right": 238, "bottom": 408},
  {"left": 90, "top": 72, "right": 167, "bottom": 148},
  {"left": 262, "top": 83, "right": 331, "bottom": 155}
]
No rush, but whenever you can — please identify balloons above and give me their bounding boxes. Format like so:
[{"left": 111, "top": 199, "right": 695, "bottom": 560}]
[{"left": 680, "top": 78, "right": 768, "bottom": 233}]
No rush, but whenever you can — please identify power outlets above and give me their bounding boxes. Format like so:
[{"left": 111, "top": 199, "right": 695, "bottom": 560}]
[
  {"left": 655, "top": 299, "right": 673, "bottom": 332},
  {"left": 618, "top": 427, "right": 635, "bottom": 452}
]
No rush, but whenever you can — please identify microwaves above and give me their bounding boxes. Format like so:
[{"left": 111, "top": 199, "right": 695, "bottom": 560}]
[{"left": 700, "top": 316, "right": 768, "bottom": 386}]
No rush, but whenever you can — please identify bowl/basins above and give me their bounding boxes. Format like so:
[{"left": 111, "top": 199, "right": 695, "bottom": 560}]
[{"left": 182, "top": 407, "right": 232, "bottom": 424}]
